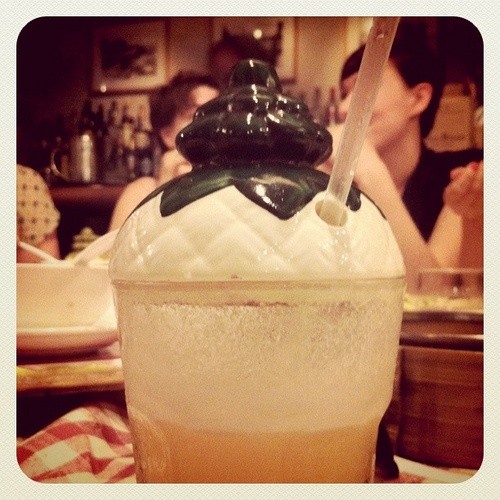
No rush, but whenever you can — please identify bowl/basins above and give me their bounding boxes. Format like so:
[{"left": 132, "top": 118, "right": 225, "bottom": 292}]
[
  {"left": 15, "top": 261, "right": 118, "bottom": 358},
  {"left": 386, "top": 308, "right": 484, "bottom": 467}
]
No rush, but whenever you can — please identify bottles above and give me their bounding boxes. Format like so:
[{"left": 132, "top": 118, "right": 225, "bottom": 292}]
[{"left": 38, "top": 98, "right": 153, "bottom": 185}]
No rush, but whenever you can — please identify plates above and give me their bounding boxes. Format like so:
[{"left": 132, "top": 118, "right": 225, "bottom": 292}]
[{"left": 16, "top": 342, "right": 126, "bottom": 395}]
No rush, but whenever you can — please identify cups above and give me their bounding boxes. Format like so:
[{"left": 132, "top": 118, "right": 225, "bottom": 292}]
[
  {"left": 106, "top": 161, "right": 407, "bottom": 484},
  {"left": 418, "top": 266, "right": 483, "bottom": 311},
  {"left": 50, "top": 130, "right": 97, "bottom": 185}
]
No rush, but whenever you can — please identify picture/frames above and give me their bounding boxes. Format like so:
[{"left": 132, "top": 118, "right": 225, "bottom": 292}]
[
  {"left": 212, "top": 17, "right": 299, "bottom": 82},
  {"left": 90, "top": 19, "right": 172, "bottom": 94}
]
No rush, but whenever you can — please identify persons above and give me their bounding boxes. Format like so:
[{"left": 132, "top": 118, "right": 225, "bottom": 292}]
[
  {"left": 317, "top": 35, "right": 484, "bottom": 299},
  {"left": 108, "top": 69, "right": 222, "bottom": 234},
  {"left": 15, "top": 164, "right": 60, "bottom": 264}
]
